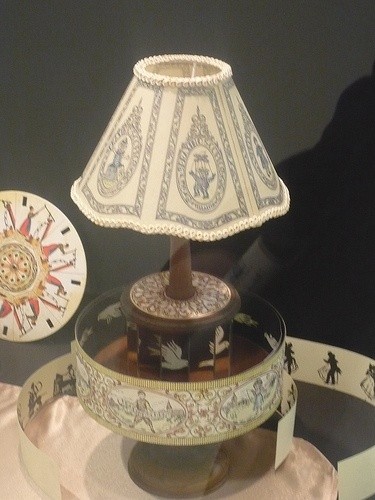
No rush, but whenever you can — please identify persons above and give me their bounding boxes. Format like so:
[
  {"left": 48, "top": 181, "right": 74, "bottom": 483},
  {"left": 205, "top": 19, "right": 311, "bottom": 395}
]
[{"left": 159, "top": 63, "right": 374, "bottom": 361}]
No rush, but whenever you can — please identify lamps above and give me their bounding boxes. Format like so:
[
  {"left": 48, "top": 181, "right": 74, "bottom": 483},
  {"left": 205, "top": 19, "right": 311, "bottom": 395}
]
[{"left": 69, "top": 54, "right": 290, "bottom": 498}]
